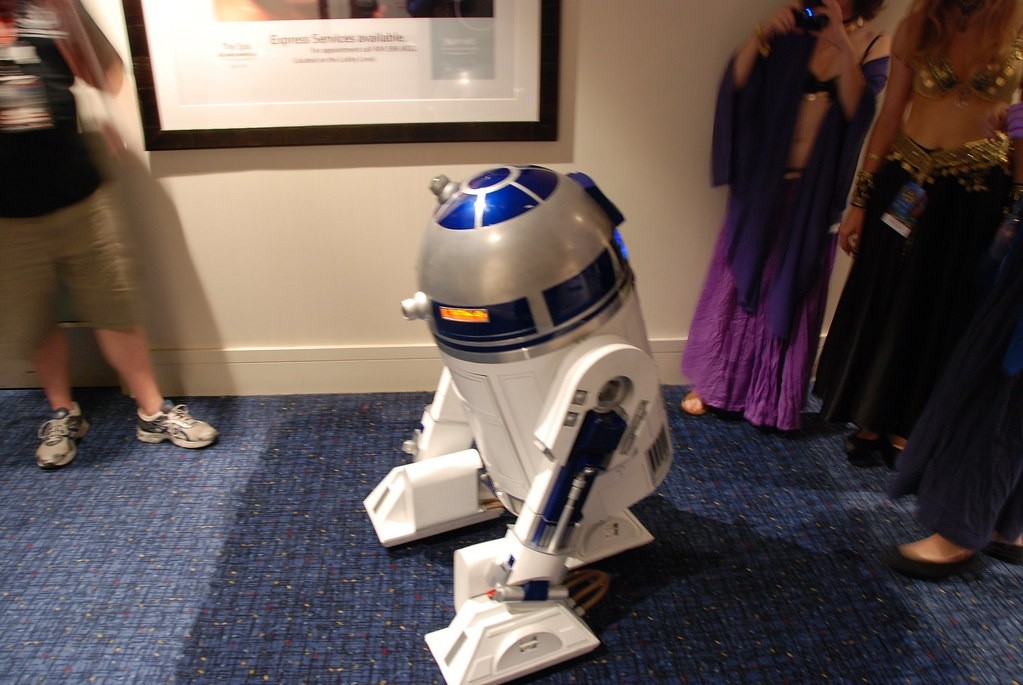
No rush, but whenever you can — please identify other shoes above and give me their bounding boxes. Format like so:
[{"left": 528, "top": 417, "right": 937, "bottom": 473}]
[
  {"left": 847, "top": 429, "right": 912, "bottom": 473},
  {"left": 682, "top": 388, "right": 710, "bottom": 414},
  {"left": 890, "top": 535, "right": 1023, "bottom": 580}
]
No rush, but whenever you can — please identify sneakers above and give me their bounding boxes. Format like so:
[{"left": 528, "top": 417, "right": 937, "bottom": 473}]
[
  {"left": 37, "top": 406, "right": 86, "bottom": 466},
  {"left": 134, "top": 401, "right": 217, "bottom": 447}
]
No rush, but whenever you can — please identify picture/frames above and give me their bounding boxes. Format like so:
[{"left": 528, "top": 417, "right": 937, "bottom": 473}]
[{"left": 120, "top": 1, "right": 562, "bottom": 151}]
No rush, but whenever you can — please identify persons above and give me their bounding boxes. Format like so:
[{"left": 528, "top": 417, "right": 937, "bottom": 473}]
[
  {"left": 814, "top": 0, "right": 1023, "bottom": 467},
  {"left": 882, "top": 104, "right": 1023, "bottom": 587},
  {"left": 677, "top": 0, "right": 889, "bottom": 431},
  {"left": 1, "top": 0, "right": 220, "bottom": 469}
]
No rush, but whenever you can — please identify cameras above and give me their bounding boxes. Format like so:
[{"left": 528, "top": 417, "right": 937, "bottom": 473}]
[{"left": 791, "top": 0, "right": 829, "bottom": 30}]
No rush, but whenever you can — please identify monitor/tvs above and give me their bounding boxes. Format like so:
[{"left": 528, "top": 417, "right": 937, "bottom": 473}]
[{"left": 124, "top": 0, "right": 561, "bottom": 153}]
